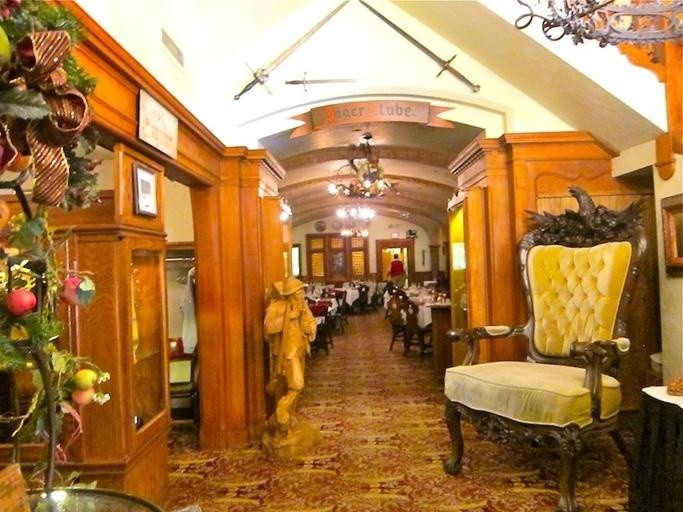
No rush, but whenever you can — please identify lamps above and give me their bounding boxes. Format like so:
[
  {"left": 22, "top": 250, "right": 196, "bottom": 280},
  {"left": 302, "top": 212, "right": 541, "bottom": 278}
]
[
  {"left": 335, "top": 202, "right": 376, "bottom": 239},
  {"left": 323, "top": 140, "right": 401, "bottom": 202}
]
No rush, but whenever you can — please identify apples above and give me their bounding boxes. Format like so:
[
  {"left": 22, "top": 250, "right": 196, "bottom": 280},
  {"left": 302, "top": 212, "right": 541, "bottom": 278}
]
[
  {"left": 73, "top": 369, "right": 96, "bottom": 390},
  {"left": 4, "top": 288, "right": 36, "bottom": 316},
  {"left": 59, "top": 276, "right": 85, "bottom": 305},
  {"left": 2, "top": 144, "right": 32, "bottom": 172},
  {"left": 72, "top": 388, "right": 95, "bottom": 405}
]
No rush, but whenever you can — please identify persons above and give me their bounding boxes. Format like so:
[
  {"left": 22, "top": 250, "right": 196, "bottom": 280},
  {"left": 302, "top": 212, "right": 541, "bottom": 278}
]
[{"left": 387, "top": 254, "right": 405, "bottom": 288}]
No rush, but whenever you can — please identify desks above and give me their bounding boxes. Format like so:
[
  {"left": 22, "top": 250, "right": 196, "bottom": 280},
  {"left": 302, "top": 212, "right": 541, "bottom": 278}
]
[{"left": 624, "top": 383, "right": 683, "bottom": 510}]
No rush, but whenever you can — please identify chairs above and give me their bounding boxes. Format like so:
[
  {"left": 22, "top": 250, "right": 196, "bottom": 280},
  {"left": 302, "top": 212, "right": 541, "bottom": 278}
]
[
  {"left": 169, "top": 353, "right": 200, "bottom": 450},
  {"left": 433, "top": 182, "right": 649, "bottom": 512}
]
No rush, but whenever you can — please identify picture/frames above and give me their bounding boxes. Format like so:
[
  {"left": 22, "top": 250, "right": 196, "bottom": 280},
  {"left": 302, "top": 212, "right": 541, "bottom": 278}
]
[
  {"left": 658, "top": 192, "right": 682, "bottom": 275},
  {"left": 132, "top": 160, "right": 157, "bottom": 219}
]
